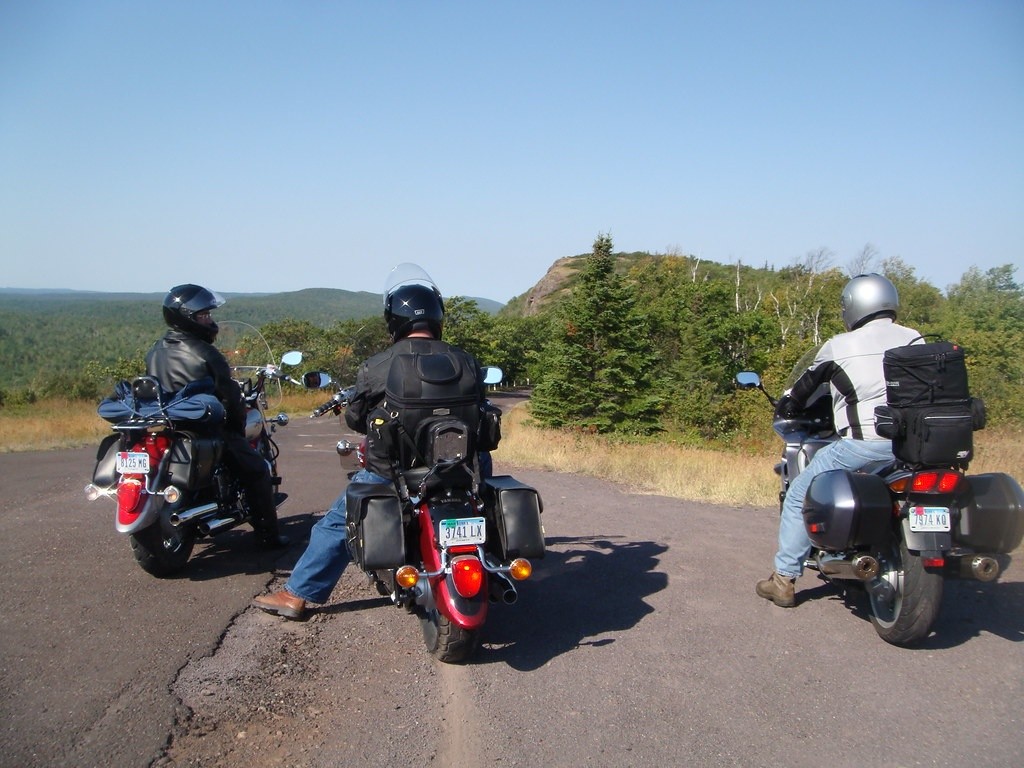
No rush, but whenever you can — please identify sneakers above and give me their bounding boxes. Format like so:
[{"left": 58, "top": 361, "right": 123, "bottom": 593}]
[
  {"left": 251, "top": 586, "right": 306, "bottom": 620},
  {"left": 754, "top": 571, "right": 797, "bottom": 607}
]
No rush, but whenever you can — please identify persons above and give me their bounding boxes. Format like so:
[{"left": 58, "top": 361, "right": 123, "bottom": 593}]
[
  {"left": 756, "top": 275, "right": 924, "bottom": 606},
  {"left": 254, "top": 286, "right": 493, "bottom": 619},
  {"left": 144, "top": 284, "right": 289, "bottom": 548}
]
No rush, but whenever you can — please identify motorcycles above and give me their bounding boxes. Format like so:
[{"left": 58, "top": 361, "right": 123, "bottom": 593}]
[
  {"left": 733, "top": 342, "right": 1024, "bottom": 648},
  {"left": 82, "top": 317, "right": 305, "bottom": 577},
  {"left": 302, "top": 317, "right": 548, "bottom": 668}
]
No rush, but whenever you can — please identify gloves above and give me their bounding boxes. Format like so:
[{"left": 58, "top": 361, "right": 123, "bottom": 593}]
[{"left": 783, "top": 396, "right": 802, "bottom": 420}]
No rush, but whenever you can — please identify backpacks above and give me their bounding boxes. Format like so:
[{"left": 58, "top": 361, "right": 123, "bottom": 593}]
[
  {"left": 873, "top": 334, "right": 993, "bottom": 471},
  {"left": 364, "top": 337, "right": 506, "bottom": 489}
]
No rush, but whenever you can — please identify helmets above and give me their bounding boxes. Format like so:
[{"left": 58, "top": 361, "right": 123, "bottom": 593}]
[
  {"left": 383, "top": 284, "right": 446, "bottom": 341},
  {"left": 839, "top": 272, "right": 899, "bottom": 329},
  {"left": 162, "top": 284, "right": 220, "bottom": 344}
]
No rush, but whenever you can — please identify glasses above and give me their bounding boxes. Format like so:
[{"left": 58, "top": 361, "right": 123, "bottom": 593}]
[{"left": 196, "top": 312, "right": 212, "bottom": 320}]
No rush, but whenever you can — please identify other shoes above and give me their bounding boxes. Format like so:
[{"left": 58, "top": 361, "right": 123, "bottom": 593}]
[{"left": 255, "top": 535, "right": 290, "bottom": 548}]
